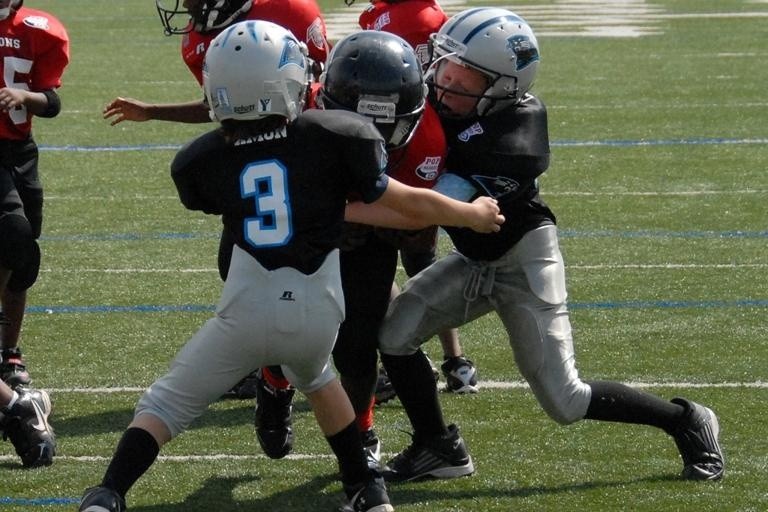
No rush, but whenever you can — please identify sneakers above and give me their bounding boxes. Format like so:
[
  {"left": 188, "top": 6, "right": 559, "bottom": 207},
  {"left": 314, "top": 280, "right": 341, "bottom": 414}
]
[
  {"left": 79, "top": 486, "right": 128, "bottom": 512},
  {"left": 3, "top": 384, "right": 55, "bottom": 469},
  {"left": 443, "top": 355, "right": 478, "bottom": 397},
  {"left": 339, "top": 425, "right": 475, "bottom": 510},
  {"left": 668, "top": 396, "right": 724, "bottom": 482},
  {"left": 375, "top": 352, "right": 441, "bottom": 405},
  {"left": 254, "top": 369, "right": 293, "bottom": 460},
  {"left": 0, "top": 349, "right": 31, "bottom": 388}
]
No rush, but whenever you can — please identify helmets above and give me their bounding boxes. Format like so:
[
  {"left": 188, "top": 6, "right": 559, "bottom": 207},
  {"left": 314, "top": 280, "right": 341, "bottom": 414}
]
[
  {"left": 200, "top": 18, "right": 309, "bottom": 122},
  {"left": 429, "top": 6, "right": 541, "bottom": 123},
  {"left": 156, "top": 0, "right": 253, "bottom": 37},
  {"left": 316, "top": 31, "right": 427, "bottom": 152}
]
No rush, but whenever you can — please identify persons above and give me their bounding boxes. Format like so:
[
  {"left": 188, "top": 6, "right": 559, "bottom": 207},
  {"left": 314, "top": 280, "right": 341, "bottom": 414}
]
[
  {"left": 345, "top": 6, "right": 724, "bottom": 482},
  {"left": 1, "top": 1, "right": 70, "bottom": 468},
  {"left": 344, "top": 0, "right": 482, "bottom": 408},
  {"left": 102, "top": 32, "right": 445, "bottom": 467},
  {"left": 102, "top": 3, "right": 333, "bottom": 402},
  {"left": 75, "top": 20, "right": 506, "bottom": 512}
]
